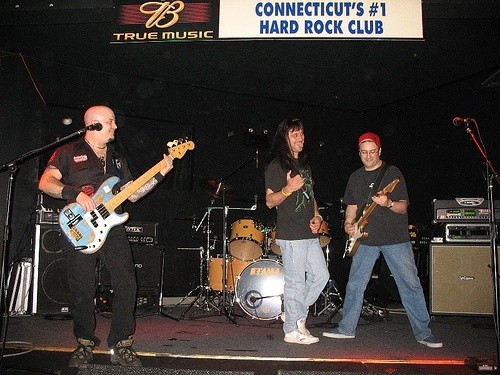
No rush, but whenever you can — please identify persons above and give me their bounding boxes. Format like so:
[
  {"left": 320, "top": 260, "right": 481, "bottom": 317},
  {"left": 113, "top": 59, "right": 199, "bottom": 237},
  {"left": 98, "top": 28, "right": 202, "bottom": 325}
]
[
  {"left": 264, "top": 119, "right": 330, "bottom": 343},
  {"left": 323, "top": 132, "right": 443, "bottom": 348},
  {"left": 38, "top": 106, "right": 173, "bottom": 366}
]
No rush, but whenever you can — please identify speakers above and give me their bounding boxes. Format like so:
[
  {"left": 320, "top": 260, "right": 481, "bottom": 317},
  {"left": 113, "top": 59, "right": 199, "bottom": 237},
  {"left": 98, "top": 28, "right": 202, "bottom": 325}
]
[
  {"left": 26, "top": 224, "right": 164, "bottom": 319},
  {"left": 426, "top": 243, "right": 500, "bottom": 317}
]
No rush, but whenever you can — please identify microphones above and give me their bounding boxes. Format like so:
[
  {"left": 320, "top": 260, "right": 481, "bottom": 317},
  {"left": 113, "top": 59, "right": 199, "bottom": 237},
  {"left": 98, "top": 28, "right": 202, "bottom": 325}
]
[
  {"left": 256, "top": 148, "right": 259, "bottom": 167},
  {"left": 86, "top": 123, "right": 102, "bottom": 131},
  {"left": 291, "top": 171, "right": 310, "bottom": 200},
  {"left": 453, "top": 117, "right": 474, "bottom": 127},
  {"left": 250, "top": 295, "right": 260, "bottom": 302}
]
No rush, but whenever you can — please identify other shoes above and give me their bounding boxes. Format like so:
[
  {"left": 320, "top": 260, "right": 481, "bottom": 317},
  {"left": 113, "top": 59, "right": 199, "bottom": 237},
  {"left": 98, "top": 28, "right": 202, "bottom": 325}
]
[
  {"left": 416, "top": 333, "right": 444, "bottom": 348},
  {"left": 322, "top": 328, "right": 356, "bottom": 338}
]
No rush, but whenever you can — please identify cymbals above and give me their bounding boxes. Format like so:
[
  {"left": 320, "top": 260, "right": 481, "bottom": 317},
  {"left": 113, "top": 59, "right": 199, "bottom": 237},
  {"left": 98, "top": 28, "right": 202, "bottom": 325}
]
[
  {"left": 199, "top": 176, "right": 230, "bottom": 200},
  {"left": 247, "top": 189, "right": 266, "bottom": 201}
]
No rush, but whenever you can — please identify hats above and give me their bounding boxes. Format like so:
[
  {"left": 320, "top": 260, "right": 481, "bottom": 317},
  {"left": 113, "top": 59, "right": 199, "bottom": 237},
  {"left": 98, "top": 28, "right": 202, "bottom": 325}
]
[{"left": 358, "top": 132, "right": 381, "bottom": 148}]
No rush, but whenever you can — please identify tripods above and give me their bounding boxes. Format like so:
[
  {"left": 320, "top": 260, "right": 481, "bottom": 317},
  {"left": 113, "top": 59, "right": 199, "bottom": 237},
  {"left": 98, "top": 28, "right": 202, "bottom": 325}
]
[{"left": 167, "top": 155, "right": 257, "bottom": 327}]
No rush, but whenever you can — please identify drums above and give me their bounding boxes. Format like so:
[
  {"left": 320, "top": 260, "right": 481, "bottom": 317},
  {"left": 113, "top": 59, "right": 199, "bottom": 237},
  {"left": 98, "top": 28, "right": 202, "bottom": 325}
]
[
  {"left": 207, "top": 254, "right": 228, "bottom": 291},
  {"left": 270, "top": 226, "right": 282, "bottom": 256},
  {"left": 234, "top": 258, "right": 285, "bottom": 321},
  {"left": 318, "top": 220, "right": 331, "bottom": 248},
  {"left": 232, "top": 258, "right": 259, "bottom": 288},
  {"left": 228, "top": 217, "right": 266, "bottom": 262}
]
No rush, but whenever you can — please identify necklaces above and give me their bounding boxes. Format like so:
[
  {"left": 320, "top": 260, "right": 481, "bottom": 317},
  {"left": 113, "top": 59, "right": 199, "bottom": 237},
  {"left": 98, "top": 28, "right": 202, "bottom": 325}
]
[{"left": 85, "top": 137, "right": 107, "bottom": 149}]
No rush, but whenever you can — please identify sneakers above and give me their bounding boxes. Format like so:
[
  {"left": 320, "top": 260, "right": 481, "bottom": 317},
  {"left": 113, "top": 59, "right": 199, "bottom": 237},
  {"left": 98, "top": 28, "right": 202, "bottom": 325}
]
[
  {"left": 68, "top": 337, "right": 95, "bottom": 368},
  {"left": 283, "top": 329, "right": 319, "bottom": 344},
  {"left": 111, "top": 335, "right": 142, "bottom": 366},
  {"left": 281, "top": 312, "right": 312, "bottom": 336}
]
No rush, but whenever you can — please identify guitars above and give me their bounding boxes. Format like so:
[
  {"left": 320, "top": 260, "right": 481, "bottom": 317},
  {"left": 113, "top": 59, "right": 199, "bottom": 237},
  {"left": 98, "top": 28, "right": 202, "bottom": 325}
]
[
  {"left": 57, "top": 136, "right": 195, "bottom": 256},
  {"left": 345, "top": 177, "right": 401, "bottom": 256}
]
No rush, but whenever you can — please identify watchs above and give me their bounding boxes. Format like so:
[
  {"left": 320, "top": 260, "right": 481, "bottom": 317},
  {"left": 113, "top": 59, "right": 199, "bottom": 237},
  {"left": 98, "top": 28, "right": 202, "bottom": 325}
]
[{"left": 388, "top": 200, "right": 394, "bottom": 209}]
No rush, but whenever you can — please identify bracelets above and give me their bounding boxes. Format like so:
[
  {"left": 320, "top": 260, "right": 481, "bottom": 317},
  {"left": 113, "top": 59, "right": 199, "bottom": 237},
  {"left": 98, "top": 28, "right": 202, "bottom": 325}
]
[
  {"left": 61, "top": 185, "right": 82, "bottom": 202},
  {"left": 153, "top": 172, "right": 164, "bottom": 182},
  {"left": 315, "top": 214, "right": 322, "bottom": 220},
  {"left": 282, "top": 186, "right": 291, "bottom": 196}
]
